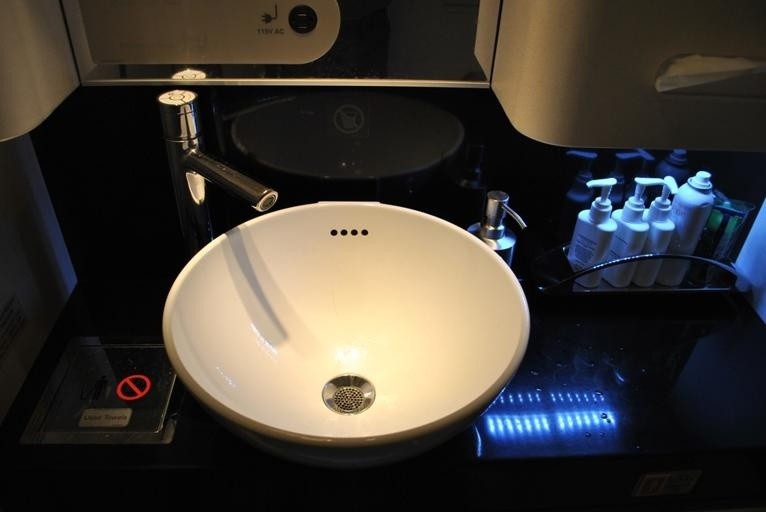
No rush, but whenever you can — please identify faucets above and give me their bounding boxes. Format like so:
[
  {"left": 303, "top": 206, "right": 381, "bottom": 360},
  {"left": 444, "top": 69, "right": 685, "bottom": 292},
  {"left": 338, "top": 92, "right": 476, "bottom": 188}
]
[
  {"left": 153, "top": 89, "right": 281, "bottom": 240},
  {"left": 169, "top": 68, "right": 299, "bottom": 165},
  {"left": 255, "top": 85, "right": 256, "bottom": 89}
]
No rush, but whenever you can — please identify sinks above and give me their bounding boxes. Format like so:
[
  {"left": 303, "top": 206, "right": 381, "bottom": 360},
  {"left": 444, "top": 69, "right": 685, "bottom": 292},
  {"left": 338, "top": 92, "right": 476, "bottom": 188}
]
[
  {"left": 163, "top": 201, "right": 530, "bottom": 467},
  {"left": 229, "top": 89, "right": 470, "bottom": 186}
]
